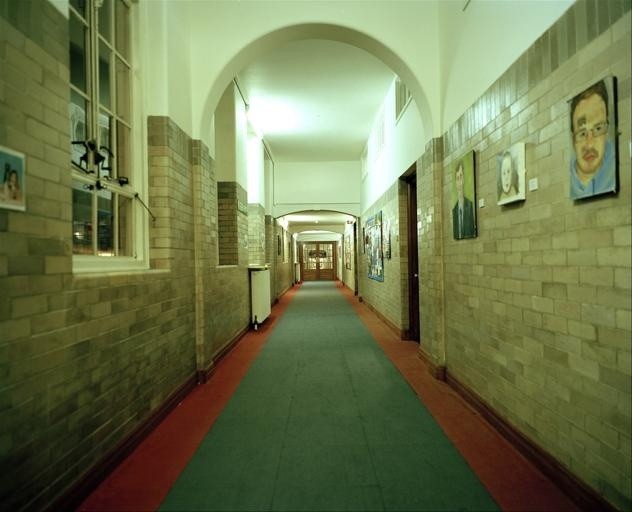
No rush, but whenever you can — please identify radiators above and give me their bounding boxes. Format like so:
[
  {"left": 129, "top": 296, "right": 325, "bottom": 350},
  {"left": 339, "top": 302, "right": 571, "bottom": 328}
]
[{"left": 249, "top": 265, "right": 272, "bottom": 331}]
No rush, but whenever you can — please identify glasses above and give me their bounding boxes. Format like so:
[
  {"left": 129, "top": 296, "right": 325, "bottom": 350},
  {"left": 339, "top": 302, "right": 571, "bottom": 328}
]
[{"left": 574, "top": 122, "right": 609, "bottom": 144}]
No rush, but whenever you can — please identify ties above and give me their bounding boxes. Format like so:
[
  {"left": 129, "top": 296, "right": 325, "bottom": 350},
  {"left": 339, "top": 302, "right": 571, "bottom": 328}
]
[{"left": 459, "top": 208, "right": 463, "bottom": 237}]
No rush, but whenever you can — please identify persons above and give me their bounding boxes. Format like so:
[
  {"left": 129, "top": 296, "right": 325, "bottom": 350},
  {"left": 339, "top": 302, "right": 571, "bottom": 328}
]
[
  {"left": 496, "top": 150, "right": 520, "bottom": 204},
  {"left": 451, "top": 161, "right": 476, "bottom": 239},
  {"left": 4, "top": 169, "right": 19, "bottom": 200},
  {"left": 568, "top": 79, "right": 613, "bottom": 200}
]
